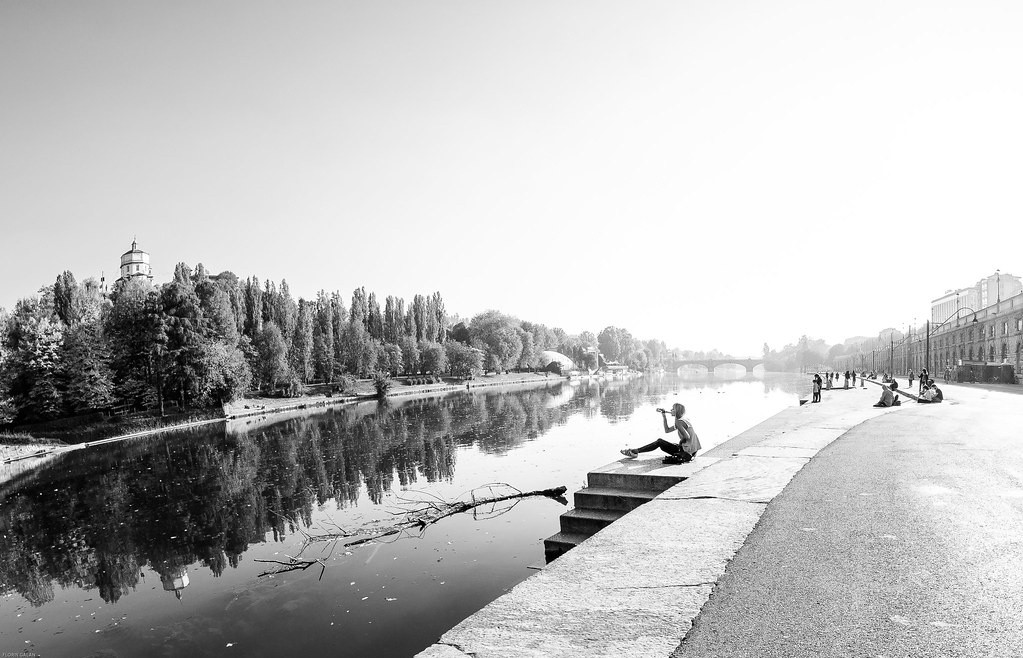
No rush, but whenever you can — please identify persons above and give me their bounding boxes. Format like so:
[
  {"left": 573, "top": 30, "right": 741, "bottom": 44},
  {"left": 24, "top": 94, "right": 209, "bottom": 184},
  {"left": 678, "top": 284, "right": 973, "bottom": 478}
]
[
  {"left": 812, "top": 380, "right": 820, "bottom": 403},
  {"left": 619, "top": 402, "right": 701, "bottom": 462},
  {"left": 825, "top": 367, "right": 957, "bottom": 408},
  {"left": 814, "top": 374, "right": 822, "bottom": 402}
]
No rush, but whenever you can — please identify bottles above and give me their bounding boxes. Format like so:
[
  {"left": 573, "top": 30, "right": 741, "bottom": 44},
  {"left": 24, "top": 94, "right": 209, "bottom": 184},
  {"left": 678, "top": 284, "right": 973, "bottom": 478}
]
[{"left": 656, "top": 408, "right": 671, "bottom": 413}]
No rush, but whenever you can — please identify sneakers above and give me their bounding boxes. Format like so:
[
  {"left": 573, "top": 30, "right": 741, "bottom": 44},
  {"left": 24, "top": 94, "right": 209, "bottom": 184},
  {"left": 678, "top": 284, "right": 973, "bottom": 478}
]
[{"left": 620, "top": 449, "right": 638, "bottom": 457}]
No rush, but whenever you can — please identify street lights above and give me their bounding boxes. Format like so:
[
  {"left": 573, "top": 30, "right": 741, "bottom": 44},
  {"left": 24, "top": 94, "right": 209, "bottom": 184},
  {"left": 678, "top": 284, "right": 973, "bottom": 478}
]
[
  {"left": 842, "top": 333, "right": 912, "bottom": 380},
  {"left": 925, "top": 306, "right": 979, "bottom": 379}
]
